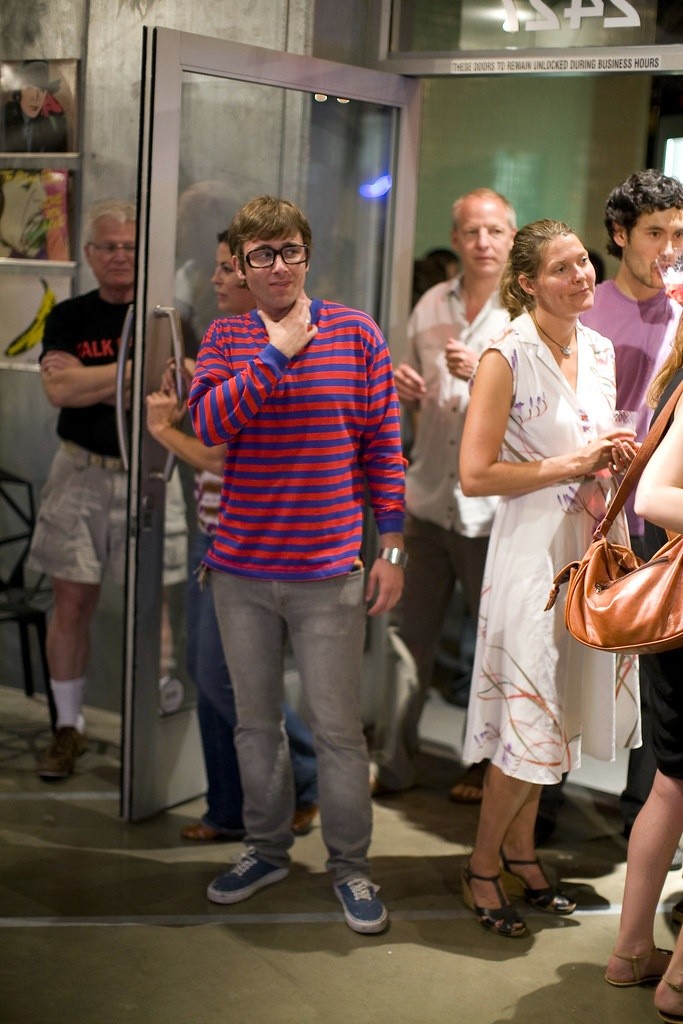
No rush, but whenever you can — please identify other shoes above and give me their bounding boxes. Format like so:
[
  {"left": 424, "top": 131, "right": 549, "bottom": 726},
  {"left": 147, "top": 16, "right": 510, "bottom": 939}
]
[
  {"left": 288, "top": 802, "right": 323, "bottom": 835},
  {"left": 623, "top": 820, "right": 682, "bottom": 872},
  {"left": 38, "top": 723, "right": 79, "bottom": 783},
  {"left": 180, "top": 819, "right": 245, "bottom": 844},
  {"left": 446, "top": 763, "right": 488, "bottom": 805}
]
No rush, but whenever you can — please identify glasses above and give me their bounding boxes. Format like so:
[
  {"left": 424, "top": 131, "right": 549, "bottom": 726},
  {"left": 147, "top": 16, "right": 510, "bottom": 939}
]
[
  {"left": 89, "top": 243, "right": 136, "bottom": 259},
  {"left": 241, "top": 240, "right": 309, "bottom": 268}
]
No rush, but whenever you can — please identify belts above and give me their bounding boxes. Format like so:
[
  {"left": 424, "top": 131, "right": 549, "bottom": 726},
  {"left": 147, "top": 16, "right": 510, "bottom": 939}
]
[{"left": 62, "top": 442, "right": 126, "bottom": 473}]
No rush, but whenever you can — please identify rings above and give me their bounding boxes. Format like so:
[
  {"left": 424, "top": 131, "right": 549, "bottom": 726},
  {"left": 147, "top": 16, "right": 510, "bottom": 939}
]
[{"left": 305, "top": 320, "right": 310, "bottom": 324}]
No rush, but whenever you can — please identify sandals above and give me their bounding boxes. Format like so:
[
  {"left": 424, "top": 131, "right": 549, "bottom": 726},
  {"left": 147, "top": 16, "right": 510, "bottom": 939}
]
[
  {"left": 652, "top": 975, "right": 683, "bottom": 1024},
  {"left": 604, "top": 947, "right": 674, "bottom": 987}
]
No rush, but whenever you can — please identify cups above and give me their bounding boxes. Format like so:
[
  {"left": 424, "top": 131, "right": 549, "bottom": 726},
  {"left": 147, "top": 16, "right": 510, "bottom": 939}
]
[
  {"left": 609, "top": 409, "right": 639, "bottom": 441},
  {"left": 655, "top": 247, "right": 683, "bottom": 307}
]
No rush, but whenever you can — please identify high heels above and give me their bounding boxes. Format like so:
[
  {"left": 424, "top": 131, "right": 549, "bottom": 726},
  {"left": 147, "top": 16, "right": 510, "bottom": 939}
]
[
  {"left": 497, "top": 847, "right": 576, "bottom": 914},
  {"left": 461, "top": 856, "right": 527, "bottom": 938}
]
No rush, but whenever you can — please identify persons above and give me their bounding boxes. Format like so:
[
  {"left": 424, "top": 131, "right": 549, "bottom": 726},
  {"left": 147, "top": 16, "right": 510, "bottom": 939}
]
[
  {"left": 145, "top": 167, "right": 683, "bottom": 1023},
  {"left": 26, "top": 201, "right": 138, "bottom": 781}
]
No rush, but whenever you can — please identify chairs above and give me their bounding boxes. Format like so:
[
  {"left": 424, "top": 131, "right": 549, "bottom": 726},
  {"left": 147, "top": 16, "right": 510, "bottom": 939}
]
[{"left": 0, "top": 467, "right": 59, "bottom": 734}]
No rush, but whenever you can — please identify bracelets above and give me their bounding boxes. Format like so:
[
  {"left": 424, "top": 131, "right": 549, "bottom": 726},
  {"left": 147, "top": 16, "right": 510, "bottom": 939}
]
[{"left": 381, "top": 548, "right": 410, "bottom": 569}]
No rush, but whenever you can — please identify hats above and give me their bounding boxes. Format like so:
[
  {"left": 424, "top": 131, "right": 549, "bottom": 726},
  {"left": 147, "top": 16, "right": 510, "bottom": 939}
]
[{"left": 13, "top": 60, "right": 62, "bottom": 95}]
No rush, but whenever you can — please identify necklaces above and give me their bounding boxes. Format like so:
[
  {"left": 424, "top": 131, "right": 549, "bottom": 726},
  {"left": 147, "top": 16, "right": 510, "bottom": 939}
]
[{"left": 532, "top": 311, "right": 575, "bottom": 357}]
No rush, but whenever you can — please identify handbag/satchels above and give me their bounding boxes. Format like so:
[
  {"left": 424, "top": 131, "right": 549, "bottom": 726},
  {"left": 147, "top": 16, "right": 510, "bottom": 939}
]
[{"left": 544, "top": 529, "right": 682, "bottom": 656}]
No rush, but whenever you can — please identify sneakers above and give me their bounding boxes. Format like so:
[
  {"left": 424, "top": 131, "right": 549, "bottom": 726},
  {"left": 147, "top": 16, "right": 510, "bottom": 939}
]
[
  {"left": 207, "top": 845, "right": 290, "bottom": 904},
  {"left": 332, "top": 874, "right": 388, "bottom": 933}
]
